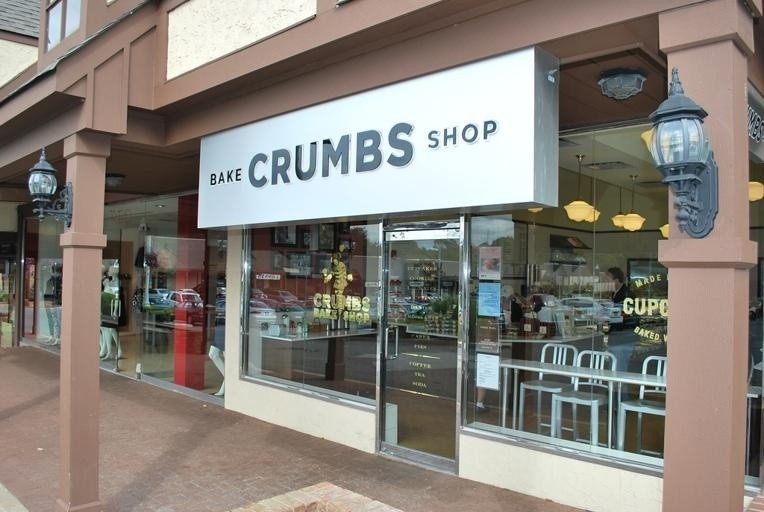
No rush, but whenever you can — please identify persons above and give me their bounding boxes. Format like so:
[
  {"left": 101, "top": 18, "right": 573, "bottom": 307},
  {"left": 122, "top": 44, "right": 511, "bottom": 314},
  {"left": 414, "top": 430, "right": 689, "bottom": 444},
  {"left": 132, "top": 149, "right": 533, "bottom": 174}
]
[
  {"left": 476, "top": 388, "right": 490, "bottom": 414},
  {"left": 606, "top": 268, "right": 638, "bottom": 417},
  {"left": 99, "top": 265, "right": 123, "bottom": 360},
  {"left": 209, "top": 318, "right": 225, "bottom": 396},
  {"left": 44, "top": 264, "right": 61, "bottom": 346}
]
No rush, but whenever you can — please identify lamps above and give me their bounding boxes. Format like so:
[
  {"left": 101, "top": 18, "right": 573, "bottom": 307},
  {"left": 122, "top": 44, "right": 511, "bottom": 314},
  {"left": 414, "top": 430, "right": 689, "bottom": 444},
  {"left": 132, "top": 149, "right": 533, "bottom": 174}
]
[
  {"left": 563, "top": 151, "right": 601, "bottom": 223},
  {"left": 610, "top": 174, "right": 645, "bottom": 231}
]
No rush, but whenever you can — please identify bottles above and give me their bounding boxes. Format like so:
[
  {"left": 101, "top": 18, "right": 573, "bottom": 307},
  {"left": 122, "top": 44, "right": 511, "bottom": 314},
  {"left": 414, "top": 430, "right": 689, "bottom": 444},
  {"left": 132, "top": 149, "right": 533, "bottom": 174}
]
[{"left": 414, "top": 261, "right": 437, "bottom": 303}]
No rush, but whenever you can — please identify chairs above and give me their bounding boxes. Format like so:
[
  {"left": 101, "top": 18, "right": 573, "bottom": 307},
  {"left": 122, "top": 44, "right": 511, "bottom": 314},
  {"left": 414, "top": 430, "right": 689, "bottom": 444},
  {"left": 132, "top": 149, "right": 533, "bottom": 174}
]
[
  {"left": 616, "top": 355, "right": 667, "bottom": 450},
  {"left": 518, "top": 342, "right": 579, "bottom": 441},
  {"left": 550, "top": 350, "right": 616, "bottom": 447}
]
